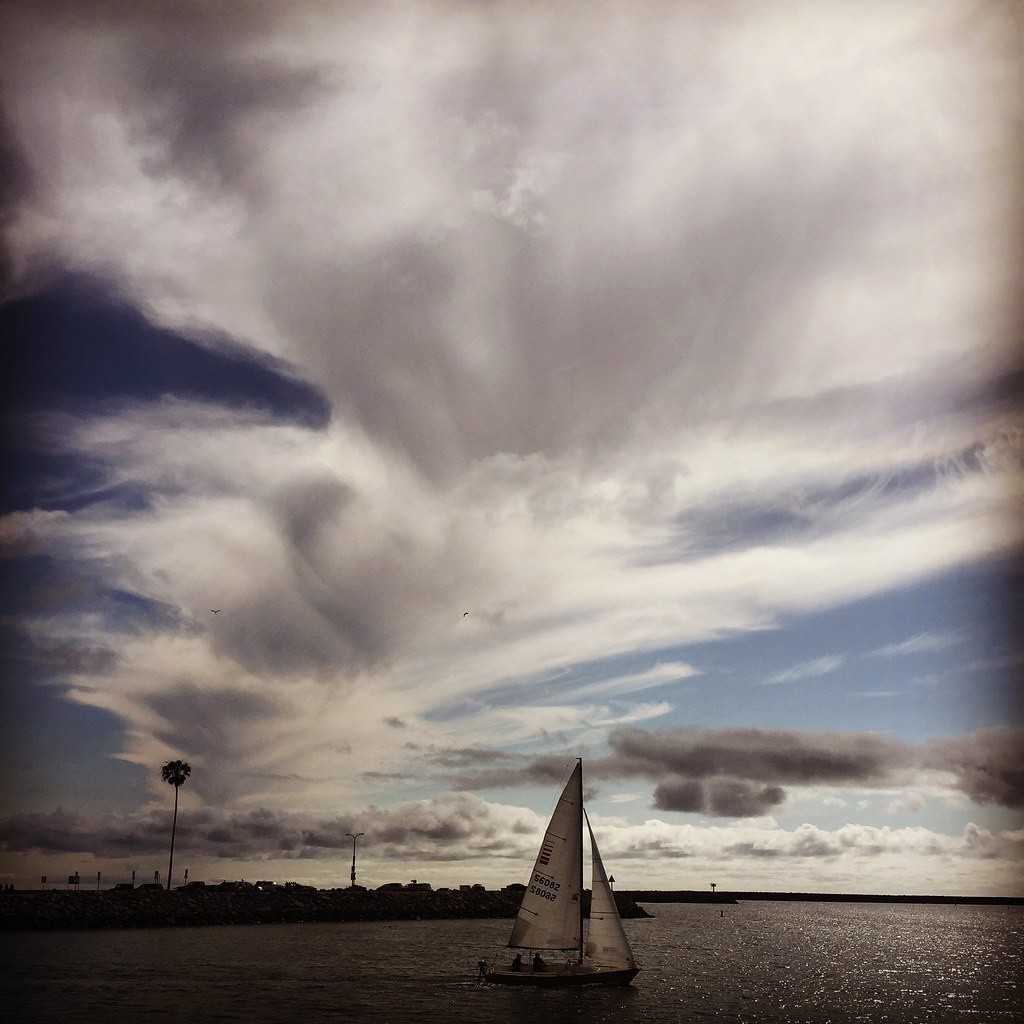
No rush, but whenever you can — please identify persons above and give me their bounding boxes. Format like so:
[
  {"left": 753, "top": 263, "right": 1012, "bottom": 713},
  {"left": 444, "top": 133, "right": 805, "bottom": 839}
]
[
  {"left": 511, "top": 953, "right": 523, "bottom": 972},
  {"left": 533, "top": 952, "right": 548, "bottom": 972}
]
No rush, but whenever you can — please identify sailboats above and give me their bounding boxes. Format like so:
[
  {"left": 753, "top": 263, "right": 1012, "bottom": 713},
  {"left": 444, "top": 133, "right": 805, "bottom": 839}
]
[{"left": 473, "top": 757, "right": 643, "bottom": 988}]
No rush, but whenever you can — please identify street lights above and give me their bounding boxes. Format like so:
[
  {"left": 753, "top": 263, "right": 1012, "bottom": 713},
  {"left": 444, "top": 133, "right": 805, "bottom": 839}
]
[{"left": 346, "top": 832, "right": 365, "bottom": 885}]
